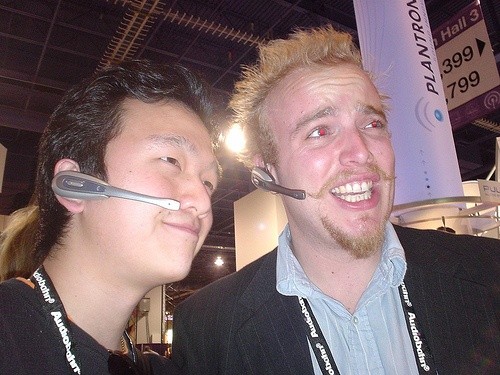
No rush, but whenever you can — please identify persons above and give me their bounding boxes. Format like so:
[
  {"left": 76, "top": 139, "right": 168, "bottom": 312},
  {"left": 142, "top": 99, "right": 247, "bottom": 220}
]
[
  {"left": 118, "top": 23, "right": 500, "bottom": 375},
  {"left": 0, "top": 57, "right": 222, "bottom": 375}
]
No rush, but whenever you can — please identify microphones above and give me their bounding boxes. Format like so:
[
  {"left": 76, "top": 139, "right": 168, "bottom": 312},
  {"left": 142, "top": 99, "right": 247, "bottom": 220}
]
[
  {"left": 251, "top": 168, "right": 305, "bottom": 201},
  {"left": 51, "top": 173, "right": 181, "bottom": 212}
]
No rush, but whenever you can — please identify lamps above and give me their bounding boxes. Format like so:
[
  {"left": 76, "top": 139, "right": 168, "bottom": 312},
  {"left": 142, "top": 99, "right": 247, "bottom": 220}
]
[{"left": 214, "top": 257, "right": 225, "bottom": 266}]
